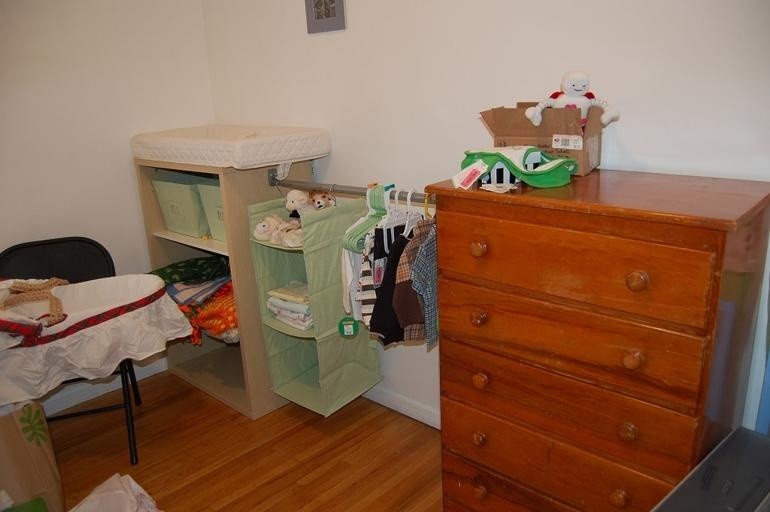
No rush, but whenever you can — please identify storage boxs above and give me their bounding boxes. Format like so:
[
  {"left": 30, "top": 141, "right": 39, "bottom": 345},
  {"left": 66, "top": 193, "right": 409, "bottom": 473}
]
[
  {"left": 480, "top": 102, "right": 619, "bottom": 177},
  {"left": 151, "top": 178, "right": 208, "bottom": 239},
  {"left": 196, "top": 183, "right": 227, "bottom": 242},
  {"left": 1, "top": 401, "right": 66, "bottom": 512}
]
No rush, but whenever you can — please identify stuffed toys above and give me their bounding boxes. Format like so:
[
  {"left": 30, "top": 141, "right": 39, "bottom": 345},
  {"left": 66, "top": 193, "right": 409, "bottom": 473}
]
[
  {"left": 283, "top": 190, "right": 335, "bottom": 252},
  {"left": 524, "top": 69, "right": 621, "bottom": 131},
  {"left": 252, "top": 189, "right": 306, "bottom": 244}
]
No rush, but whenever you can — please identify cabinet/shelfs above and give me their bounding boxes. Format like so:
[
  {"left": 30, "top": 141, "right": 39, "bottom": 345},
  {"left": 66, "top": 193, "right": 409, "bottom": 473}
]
[
  {"left": 130, "top": 138, "right": 314, "bottom": 421},
  {"left": 424, "top": 184, "right": 770, "bottom": 512}
]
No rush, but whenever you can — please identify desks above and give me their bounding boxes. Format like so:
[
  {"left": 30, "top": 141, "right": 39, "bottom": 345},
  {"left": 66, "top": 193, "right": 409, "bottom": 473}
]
[{"left": 0, "top": 273, "right": 166, "bottom": 406}]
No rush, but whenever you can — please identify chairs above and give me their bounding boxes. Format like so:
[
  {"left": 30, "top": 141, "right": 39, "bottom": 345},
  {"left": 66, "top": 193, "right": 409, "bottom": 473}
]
[{"left": 0, "top": 236, "right": 142, "bottom": 465}]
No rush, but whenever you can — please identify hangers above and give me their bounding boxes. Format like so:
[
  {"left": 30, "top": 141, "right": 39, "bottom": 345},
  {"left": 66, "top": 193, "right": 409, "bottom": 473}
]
[{"left": 341, "top": 185, "right": 432, "bottom": 254}]
